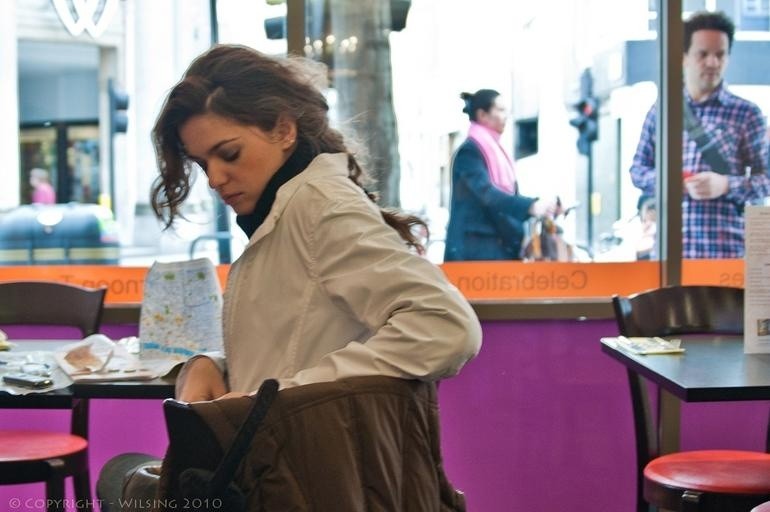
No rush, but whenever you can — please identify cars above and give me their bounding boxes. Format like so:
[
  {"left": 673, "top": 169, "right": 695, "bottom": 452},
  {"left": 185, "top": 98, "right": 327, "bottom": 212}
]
[
  {"left": 399, "top": 201, "right": 449, "bottom": 265},
  {"left": 592, "top": 227, "right": 658, "bottom": 263}
]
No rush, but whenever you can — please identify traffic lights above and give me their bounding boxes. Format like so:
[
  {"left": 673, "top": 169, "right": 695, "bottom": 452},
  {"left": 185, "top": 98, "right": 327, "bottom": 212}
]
[
  {"left": 265, "top": 1, "right": 315, "bottom": 69},
  {"left": 110, "top": 91, "right": 129, "bottom": 134},
  {"left": 568, "top": 96, "right": 599, "bottom": 142}
]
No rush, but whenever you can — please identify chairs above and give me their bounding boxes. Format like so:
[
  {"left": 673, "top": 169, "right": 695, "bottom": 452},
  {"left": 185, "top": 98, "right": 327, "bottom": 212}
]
[
  {"left": 610, "top": 283, "right": 770, "bottom": 512},
  {"left": 160, "top": 378, "right": 448, "bottom": 512},
  {"left": 1, "top": 280, "right": 109, "bottom": 512}
]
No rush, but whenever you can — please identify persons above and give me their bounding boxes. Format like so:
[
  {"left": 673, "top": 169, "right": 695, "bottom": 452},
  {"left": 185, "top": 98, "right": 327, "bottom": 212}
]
[
  {"left": 444, "top": 88, "right": 554, "bottom": 261},
  {"left": 30, "top": 168, "right": 57, "bottom": 206},
  {"left": 627, "top": 11, "right": 769, "bottom": 259},
  {"left": 95, "top": 44, "right": 485, "bottom": 511}
]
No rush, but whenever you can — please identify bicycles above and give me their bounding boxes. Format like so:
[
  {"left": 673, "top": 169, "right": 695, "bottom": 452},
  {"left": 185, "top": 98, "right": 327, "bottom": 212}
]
[{"left": 520, "top": 195, "right": 595, "bottom": 262}]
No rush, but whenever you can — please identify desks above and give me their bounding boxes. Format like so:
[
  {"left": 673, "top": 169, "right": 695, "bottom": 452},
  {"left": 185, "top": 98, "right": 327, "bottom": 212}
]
[
  {"left": 1, "top": 334, "right": 229, "bottom": 399},
  {"left": 597, "top": 329, "right": 770, "bottom": 402}
]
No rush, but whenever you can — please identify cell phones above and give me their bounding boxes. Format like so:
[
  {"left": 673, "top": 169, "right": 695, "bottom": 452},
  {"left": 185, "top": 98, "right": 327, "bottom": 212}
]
[{"left": 3, "top": 372, "right": 53, "bottom": 389}]
